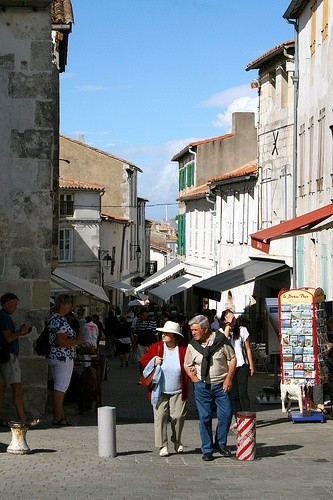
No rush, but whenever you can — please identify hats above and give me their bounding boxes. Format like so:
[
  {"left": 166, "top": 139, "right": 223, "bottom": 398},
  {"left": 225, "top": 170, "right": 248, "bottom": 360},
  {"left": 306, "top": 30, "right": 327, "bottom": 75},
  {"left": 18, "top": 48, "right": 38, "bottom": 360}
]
[{"left": 156, "top": 320, "right": 184, "bottom": 338}]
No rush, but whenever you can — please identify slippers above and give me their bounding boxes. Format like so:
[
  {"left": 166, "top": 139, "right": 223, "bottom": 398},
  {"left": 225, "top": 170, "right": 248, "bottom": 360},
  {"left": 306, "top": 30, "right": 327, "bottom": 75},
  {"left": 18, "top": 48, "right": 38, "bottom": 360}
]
[{"left": 52, "top": 418, "right": 73, "bottom": 425}]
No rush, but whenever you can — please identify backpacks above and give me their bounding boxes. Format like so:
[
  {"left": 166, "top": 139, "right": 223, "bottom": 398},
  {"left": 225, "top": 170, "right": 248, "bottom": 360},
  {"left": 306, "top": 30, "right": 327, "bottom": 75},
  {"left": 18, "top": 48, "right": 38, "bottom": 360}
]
[{"left": 34, "top": 328, "right": 51, "bottom": 356}]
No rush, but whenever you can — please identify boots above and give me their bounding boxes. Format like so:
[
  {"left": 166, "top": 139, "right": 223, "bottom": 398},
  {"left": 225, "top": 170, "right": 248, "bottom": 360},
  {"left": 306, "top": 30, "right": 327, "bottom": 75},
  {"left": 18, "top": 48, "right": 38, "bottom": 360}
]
[
  {"left": 125, "top": 361, "right": 128, "bottom": 367},
  {"left": 120, "top": 361, "right": 124, "bottom": 367}
]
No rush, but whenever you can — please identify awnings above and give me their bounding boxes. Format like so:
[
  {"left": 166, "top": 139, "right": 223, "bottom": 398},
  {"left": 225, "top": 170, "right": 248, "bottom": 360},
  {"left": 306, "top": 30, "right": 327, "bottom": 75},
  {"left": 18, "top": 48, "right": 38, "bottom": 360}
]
[
  {"left": 192, "top": 260, "right": 292, "bottom": 301},
  {"left": 134, "top": 258, "right": 186, "bottom": 292},
  {"left": 110, "top": 281, "right": 145, "bottom": 300},
  {"left": 52, "top": 269, "right": 110, "bottom": 303},
  {"left": 149, "top": 273, "right": 203, "bottom": 301},
  {"left": 50, "top": 275, "right": 83, "bottom": 292}
]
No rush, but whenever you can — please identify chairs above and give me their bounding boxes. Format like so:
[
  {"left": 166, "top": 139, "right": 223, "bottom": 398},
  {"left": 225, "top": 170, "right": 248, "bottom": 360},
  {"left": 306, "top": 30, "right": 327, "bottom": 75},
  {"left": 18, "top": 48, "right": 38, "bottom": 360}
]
[{"left": 250, "top": 339, "right": 270, "bottom": 373}]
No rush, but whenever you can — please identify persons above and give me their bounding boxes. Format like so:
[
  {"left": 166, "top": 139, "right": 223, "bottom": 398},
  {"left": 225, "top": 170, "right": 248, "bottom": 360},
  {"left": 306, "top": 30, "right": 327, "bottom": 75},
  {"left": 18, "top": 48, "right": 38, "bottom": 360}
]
[
  {"left": 46, "top": 294, "right": 79, "bottom": 427},
  {"left": 325, "top": 315, "right": 333, "bottom": 416},
  {"left": 68, "top": 302, "right": 223, "bottom": 385},
  {"left": 220, "top": 309, "right": 255, "bottom": 426},
  {"left": 141, "top": 320, "right": 197, "bottom": 458},
  {"left": 0, "top": 293, "right": 44, "bottom": 426},
  {"left": 184, "top": 315, "right": 236, "bottom": 460}
]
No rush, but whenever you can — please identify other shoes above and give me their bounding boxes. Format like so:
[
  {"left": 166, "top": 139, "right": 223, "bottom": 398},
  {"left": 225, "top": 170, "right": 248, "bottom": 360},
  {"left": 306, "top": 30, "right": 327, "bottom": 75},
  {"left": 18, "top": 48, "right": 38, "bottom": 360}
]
[
  {"left": 202, "top": 453, "right": 214, "bottom": 461},
  {"left": 25, "top": 418, "right": 41, "bottom": 428},
  {"left": 171, "top": 436, "right": 183, "bottom": 453},
  {"left": 214, "top": 443, "right": 231, "bottom": 456},
  {"left": 158, "top": 443, "right": 169, "bottom": 457},
  {"left": 137, "top": 380, "right": 142, "bottom": 385}
]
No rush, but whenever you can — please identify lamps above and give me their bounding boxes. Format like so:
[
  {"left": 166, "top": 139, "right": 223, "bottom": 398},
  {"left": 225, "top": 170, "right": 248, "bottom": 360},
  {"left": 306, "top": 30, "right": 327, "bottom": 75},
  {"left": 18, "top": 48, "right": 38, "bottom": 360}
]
[
  {"left": 99, "top": 249, "right": 115, "bottom": 271},
  {"left": 59, "top": 158, "right": 71, "bottom": 164},
  {"left": 130, "top": 244, "right": 143, "bottom": 259}
]
